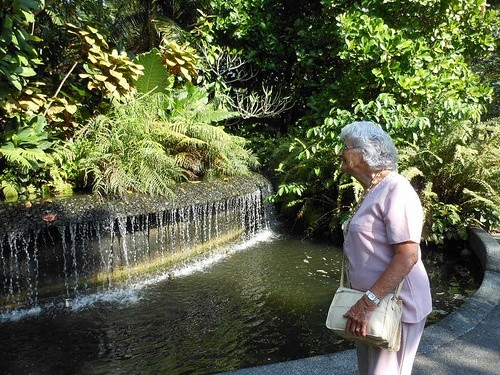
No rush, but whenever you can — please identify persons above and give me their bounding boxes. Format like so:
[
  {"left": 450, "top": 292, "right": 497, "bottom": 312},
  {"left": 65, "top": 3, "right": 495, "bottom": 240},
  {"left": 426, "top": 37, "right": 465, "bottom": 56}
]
[{"left": 340, "top": 121, "right": 432, "bottom": 375}]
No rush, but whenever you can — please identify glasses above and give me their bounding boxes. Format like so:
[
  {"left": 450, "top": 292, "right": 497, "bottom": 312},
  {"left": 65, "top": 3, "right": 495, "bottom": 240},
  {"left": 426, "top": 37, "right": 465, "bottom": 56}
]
[{"left": 339, "top": 144, "right": 359, "bottom": 150}]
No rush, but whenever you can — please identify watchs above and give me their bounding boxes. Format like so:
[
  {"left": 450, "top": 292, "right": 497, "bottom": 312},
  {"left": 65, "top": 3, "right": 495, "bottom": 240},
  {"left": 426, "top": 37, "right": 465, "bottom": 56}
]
[{"left": 363, "top": 288, "right": 380, "bottom": 303}]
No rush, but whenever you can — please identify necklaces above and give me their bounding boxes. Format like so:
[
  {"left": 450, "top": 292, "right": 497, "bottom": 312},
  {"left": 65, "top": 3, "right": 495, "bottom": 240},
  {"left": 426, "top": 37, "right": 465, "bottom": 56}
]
[{"left": 347, "top": 170, "right": 382, "bottom": 234}]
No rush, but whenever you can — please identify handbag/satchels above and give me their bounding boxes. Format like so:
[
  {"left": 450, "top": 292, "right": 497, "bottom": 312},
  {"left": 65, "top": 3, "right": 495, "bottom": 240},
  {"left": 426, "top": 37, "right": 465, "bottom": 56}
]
[{"left": 326, "top": 285, "right": 403, "bottom": 351}]
